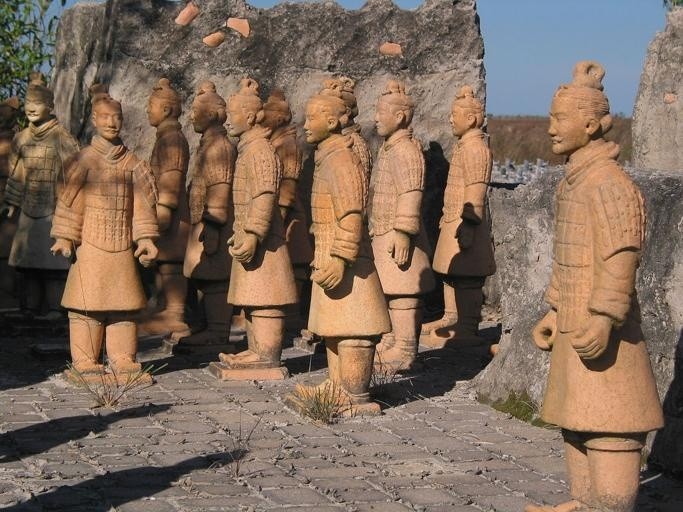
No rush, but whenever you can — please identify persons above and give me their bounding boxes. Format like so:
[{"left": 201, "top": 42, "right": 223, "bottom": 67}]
[
  {"left": 48, "top": 84, "right": 160, "bottom": 375},
  {"left": 1, "top": 95, "right": 27, "bottom": 306},
  {"left": 218, "top": 78, "right": 298, "bottom": 369},
  {"left": 322, "top": 77, "right": 372, "bottom": 191},
  {"left": 524, "top": 60, "right": 667, "bottom": 512},
  {"left": 178, "top": 82, "right": 237, "bottom": 345},
  {"left": 363, "top": 82, "right": 436, "bottom": 378},
  {"left": 301, "top": 78, "right": 395, "bottom": 404},
  {"left": 0, "top": 73, "right": 80, "bottom": 320},
  {"left": 260, "top": 86, "right": 313, "bottom": 314},
  {"left": 138, "top": 77, "right": 189, "bottom": 334},
  {"left": 431, "top": 85, "right": 492, "bottom": 339}
]
